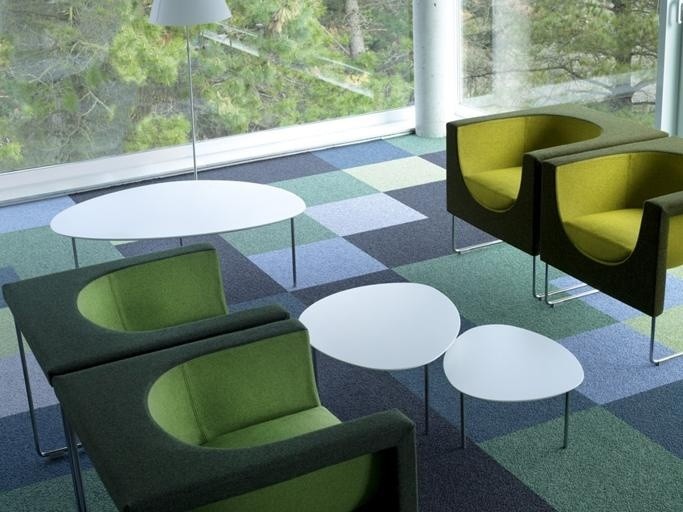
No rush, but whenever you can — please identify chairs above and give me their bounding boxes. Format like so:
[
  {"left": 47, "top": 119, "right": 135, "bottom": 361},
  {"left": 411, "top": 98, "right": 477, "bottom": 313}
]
[
  {"left": 446, "top": 103, "right": 669, "bottom": 301},
  {"left": 3, "top": 242, "right": 291, "bottom": 511},
  {"left": 54, "top": 319, "right": 420, "bottom": 512},
  {"left": 540, "top": 136, "right": 683, "bottom": 366}
]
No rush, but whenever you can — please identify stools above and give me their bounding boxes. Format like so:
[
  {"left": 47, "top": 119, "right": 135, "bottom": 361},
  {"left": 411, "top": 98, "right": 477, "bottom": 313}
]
[
  {"left": 298, "top": 283, "right": 461, "bottom": 436},
  {"left": 442, "top": 323, "right": 585, "bottom": 451}
]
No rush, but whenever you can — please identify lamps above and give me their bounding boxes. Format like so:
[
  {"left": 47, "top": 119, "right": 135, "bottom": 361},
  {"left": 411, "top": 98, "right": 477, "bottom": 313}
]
[{"left": 148, "top": 1, "right": 232, "bottom": 180}]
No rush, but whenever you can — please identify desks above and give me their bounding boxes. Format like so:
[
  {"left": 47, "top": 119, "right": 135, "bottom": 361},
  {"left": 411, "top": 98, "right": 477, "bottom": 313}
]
[{"left": 50, "top": 180, "right": 307, "bottom": 287}]
